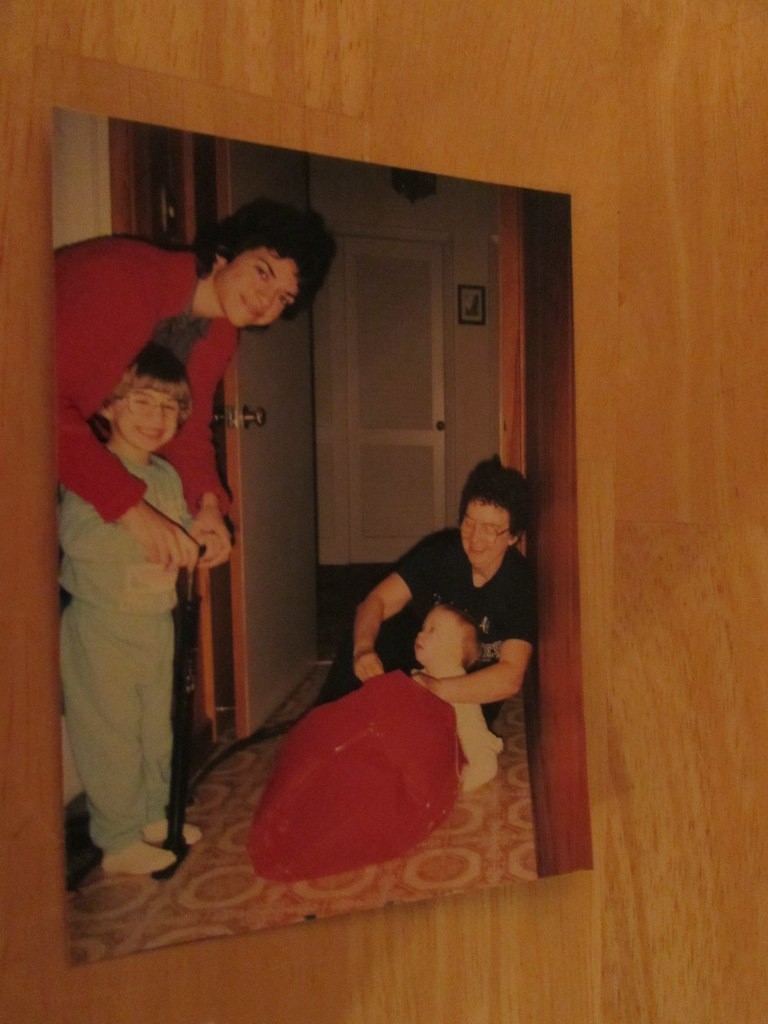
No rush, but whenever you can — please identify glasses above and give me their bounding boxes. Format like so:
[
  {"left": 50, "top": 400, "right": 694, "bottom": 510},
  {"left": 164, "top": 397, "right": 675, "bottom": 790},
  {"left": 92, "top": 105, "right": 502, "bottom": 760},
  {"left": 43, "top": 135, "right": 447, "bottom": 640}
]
[
  {"left": 117, "top": 388, "right": 184, "bottom": 419},
  {"left": 463, "top": 517, "right": 512, "bottom": 541}
]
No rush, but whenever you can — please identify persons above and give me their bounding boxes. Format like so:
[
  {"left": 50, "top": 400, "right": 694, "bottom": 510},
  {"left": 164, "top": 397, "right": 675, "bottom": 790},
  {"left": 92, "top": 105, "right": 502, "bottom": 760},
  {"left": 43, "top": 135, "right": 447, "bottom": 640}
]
[
  {"left": 50, "top": 195, "right": 333, "bottom": 884},
  {"left": 402, "top": 601, "right": 505, "bottom": 792},
  {"left": 313, "top": 456, "right": 536, "bottom": 729},
  {"left": 54, "top": 340, "right": 223, "bottom": 876}
]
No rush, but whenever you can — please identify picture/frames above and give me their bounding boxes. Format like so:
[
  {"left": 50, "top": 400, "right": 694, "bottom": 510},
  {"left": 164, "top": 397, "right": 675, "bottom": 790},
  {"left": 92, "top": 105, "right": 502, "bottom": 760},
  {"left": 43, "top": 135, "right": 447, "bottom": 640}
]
[{"left": 458, "top": 284, "right": 485, "bottom": 326}]
